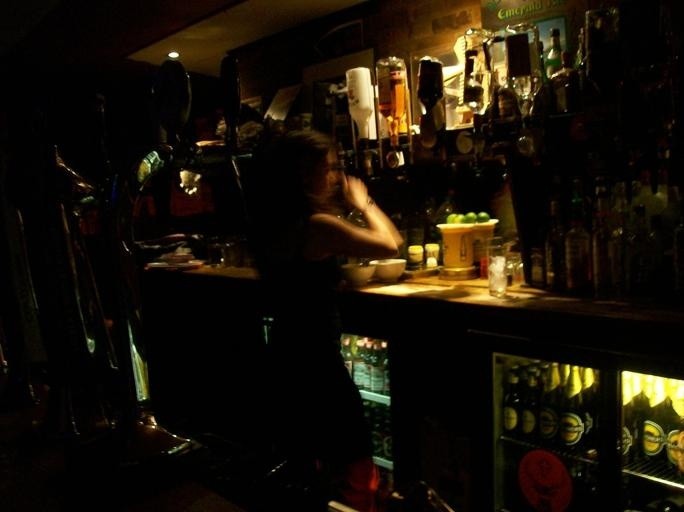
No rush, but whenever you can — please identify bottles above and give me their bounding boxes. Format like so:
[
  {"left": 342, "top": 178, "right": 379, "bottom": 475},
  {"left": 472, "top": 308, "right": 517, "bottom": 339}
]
[
  {"left": 500, "top": 22, "right": 542, "bottom": 116},
  {"left": 343, "top": 67, "right": 374, "bottom": 153},
  {"left": 521, "top": 162, "right": 683, "bottom": 293},
  {"left": 584, "top": 11, "right": 606, "bottom": 82},
  {"left": 460, "top": 26, "right": 494, "bottom": 127},
  {"left": 372, "top": 56, "right": 407, "bottom": 147},
  {"left": 543, "top": 26, "right": 564, "bottom": 72},
  {"left": 415, "top": 54, "right": 444, "bottom": 115},
  {"left": 335, "top": 335, "right": 390, "bottom": 463},
  {"left": 500, "top": 360, "right": 683, "bottom": 511}
]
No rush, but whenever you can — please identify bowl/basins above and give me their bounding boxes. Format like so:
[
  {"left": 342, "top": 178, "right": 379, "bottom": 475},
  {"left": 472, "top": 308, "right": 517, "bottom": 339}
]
[
  {"left": 341, "top": 263, "right": 375, "bottom": 288},
  {"left": 370, "top": 258, "right": 407, "bottom": 282}
]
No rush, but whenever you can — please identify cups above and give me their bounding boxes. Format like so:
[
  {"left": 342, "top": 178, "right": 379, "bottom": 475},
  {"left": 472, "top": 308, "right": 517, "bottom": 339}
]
[
  {"left": 222, "top": 243, "right": 239, "bottom": 266},
  {"left": 486, "top": 244, "right": 510, "bottom": 298},
  {"left": 425, "top": 243, "right": 440, "bottom": 262},
  {"left": 407, "top": 244, "right": 423, "bottom": 264},
  {"left": 204, "top": 234, "right": 223, "bottom": 265}
]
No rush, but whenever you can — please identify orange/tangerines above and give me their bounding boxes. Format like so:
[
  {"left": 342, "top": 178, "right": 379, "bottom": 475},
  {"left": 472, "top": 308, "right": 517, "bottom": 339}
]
[{"left": 447, "top": 212, "right": 490, "bottom": 224}]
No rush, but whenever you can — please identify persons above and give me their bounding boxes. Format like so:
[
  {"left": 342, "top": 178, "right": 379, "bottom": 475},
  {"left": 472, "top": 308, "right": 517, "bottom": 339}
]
[{"left": 247, "top": 131, "right": 405, "bottom": 491}]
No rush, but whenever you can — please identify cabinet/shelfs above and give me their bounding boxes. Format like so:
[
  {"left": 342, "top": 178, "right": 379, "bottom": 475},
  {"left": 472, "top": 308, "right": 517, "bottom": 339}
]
[
  {"left": 460, "top": 328, "right": 684, "bottom": 510},
  {"left": 256, "top": 313, "right": 396, "bottom": 498}
]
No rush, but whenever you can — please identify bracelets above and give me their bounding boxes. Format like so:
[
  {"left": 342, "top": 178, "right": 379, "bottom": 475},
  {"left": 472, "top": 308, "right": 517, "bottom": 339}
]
[{"left": 361, "top": 198, "right": 374, "bottom": 213}]
